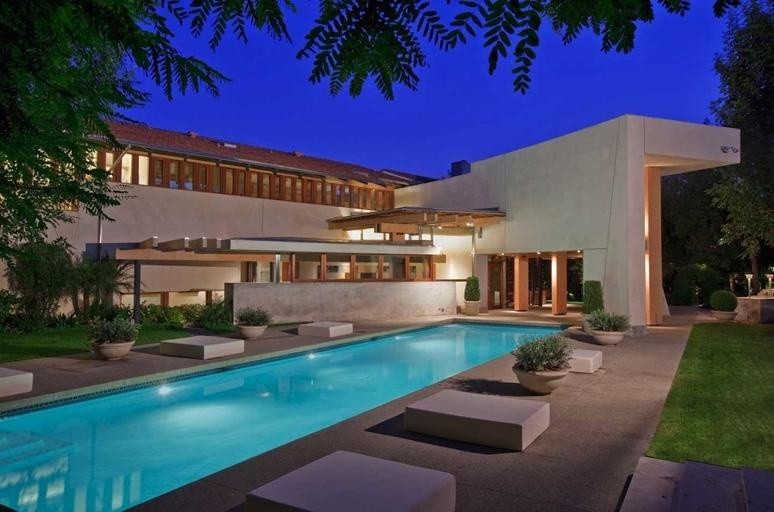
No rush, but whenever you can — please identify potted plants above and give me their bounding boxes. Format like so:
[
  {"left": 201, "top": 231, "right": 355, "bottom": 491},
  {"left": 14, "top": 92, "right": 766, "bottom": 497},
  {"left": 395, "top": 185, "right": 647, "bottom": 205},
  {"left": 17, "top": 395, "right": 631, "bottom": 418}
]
[
  {"left": 85, "top": 313, "right": 142, "bottom": 360},
  {"left": 464, "top": 276, "right": 482, "bottom": 315},
  {"left": 235, "top": 305, "right": 273, "bottom": 340},
  {"left": 711, "top": 291, "right": 738, "bottom": 321},
  {"left": 510, "top": 335, "right": 576, "bottom": 395},
  {"left": 580, "top": 279, "right": 605, "bottom": 331},
  {"left": 587, "top": 309, "right": 631, "bottom": 346}
]
